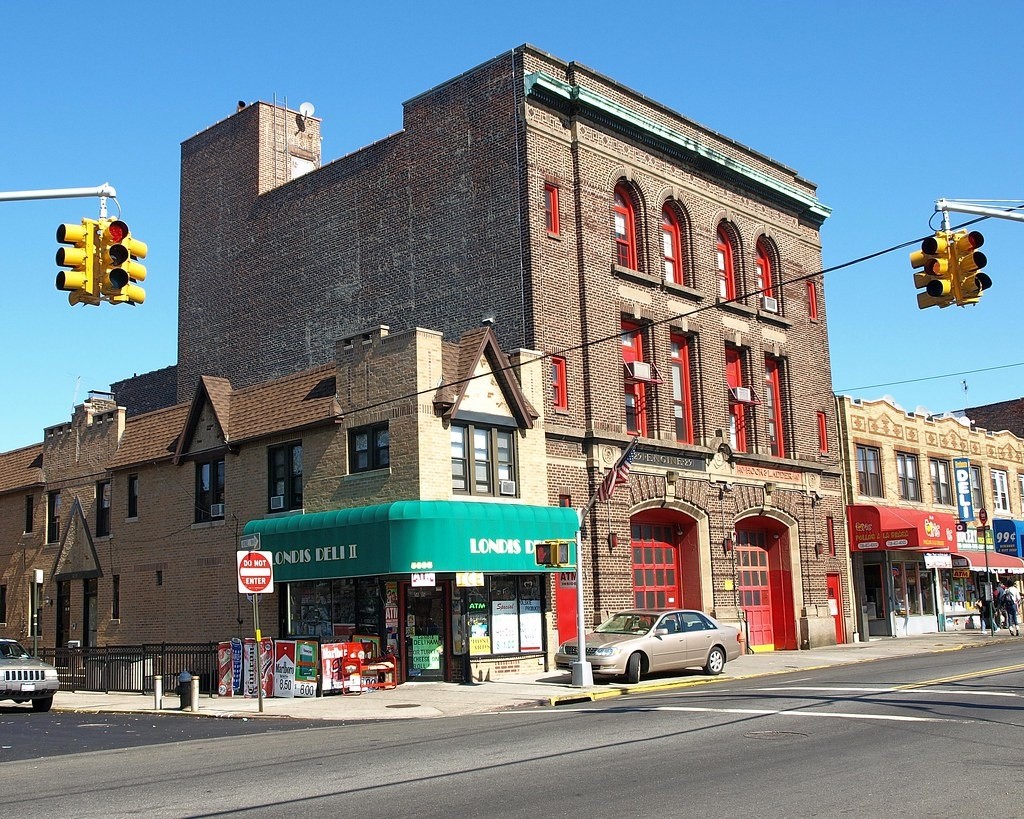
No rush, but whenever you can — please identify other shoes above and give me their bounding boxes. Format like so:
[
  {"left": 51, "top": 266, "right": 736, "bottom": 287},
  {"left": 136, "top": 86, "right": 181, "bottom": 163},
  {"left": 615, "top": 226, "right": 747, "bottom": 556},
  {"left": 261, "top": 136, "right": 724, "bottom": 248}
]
[
  {"left": 1016, "top": 630, "right": 1020, "bottom": 636},
  {"left": 1004, "top": 626, "right": 1008, "bottom": 629},
  {"left": 981, "top": 631, "right": 987, "bottom": 634},
  {"left": 994, "top": 628, "right": 1000, "bottom": 633},
  {"left": 999, "top": 626, "right": 1003, "bottom": 629},
  {"left": 1010, "top": 630, "right": 1014, "bottom": 636}
]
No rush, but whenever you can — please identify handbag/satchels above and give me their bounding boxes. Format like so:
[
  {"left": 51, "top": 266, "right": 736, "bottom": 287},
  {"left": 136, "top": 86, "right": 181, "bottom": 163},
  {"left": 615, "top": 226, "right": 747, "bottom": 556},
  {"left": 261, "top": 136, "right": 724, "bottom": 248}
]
[
  {"left": 1000, "top": 615, "right": 1005, "bottom": 623},
  {"left": 976, "top": 600, "right": 982, "bottom": 607}
]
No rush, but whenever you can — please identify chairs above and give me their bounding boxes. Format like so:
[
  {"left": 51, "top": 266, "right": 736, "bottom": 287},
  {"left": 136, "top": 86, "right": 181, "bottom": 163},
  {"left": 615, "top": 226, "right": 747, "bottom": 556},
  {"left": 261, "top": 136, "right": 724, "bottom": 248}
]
[{"left": 341, "top": 641, "right": 397, "bottom": 696}]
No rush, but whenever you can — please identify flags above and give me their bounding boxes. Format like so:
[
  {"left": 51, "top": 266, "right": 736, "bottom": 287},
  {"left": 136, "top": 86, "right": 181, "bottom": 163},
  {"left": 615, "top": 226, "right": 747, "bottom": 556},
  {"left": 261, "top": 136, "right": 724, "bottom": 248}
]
[{"left": 597, "top": 439, "right": 637, "bottom": 501}]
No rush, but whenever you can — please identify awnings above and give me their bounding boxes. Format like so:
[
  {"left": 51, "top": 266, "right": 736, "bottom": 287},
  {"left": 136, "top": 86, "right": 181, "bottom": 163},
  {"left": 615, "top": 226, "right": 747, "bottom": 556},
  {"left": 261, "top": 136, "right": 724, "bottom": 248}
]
[
  {"left": 951, "top": 551, "right": 1024, "bottom": 574},
  {"left": 846, "top": 504, "right": 958, "bottom": 553},
  {"left": 243, "top": 500, "right": 581, "bottom": 583},
  {"left": 992, "top": 518, "right": 1024, "bottom": 557}
]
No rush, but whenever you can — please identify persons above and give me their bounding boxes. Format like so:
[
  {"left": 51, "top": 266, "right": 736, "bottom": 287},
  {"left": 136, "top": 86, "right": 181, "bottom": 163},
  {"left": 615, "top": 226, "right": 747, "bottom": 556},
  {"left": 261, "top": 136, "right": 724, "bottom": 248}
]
[{"left": 994, "top": 579, "right": 1021, "bottom": 636}]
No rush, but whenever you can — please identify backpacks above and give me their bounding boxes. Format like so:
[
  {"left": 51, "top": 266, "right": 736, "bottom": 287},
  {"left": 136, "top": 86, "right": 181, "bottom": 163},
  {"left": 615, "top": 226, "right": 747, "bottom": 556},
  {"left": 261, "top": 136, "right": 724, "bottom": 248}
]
[{"left": 1003, "top": 589, "right": 1014, "bottom": 607}]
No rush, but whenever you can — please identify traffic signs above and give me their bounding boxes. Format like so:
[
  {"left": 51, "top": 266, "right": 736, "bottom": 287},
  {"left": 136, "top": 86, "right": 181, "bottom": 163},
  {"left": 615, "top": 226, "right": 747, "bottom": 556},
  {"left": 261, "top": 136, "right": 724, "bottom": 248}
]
[{"left": 237, "top": 532, "right": 260, "bottom": 551}]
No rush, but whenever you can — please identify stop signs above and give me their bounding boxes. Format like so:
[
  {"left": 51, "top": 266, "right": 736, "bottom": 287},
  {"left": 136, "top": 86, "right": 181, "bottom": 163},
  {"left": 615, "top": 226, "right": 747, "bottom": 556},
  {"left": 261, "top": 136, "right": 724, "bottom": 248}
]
[{"left": 236, "top": 552, "right": 275, "bottom": 595}]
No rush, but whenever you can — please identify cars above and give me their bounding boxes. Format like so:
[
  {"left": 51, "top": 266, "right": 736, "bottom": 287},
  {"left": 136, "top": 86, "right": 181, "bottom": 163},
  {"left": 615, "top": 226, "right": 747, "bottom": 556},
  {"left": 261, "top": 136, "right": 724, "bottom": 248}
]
[{"left": 553, "top": 608, "right": 745, "bottom": 684}]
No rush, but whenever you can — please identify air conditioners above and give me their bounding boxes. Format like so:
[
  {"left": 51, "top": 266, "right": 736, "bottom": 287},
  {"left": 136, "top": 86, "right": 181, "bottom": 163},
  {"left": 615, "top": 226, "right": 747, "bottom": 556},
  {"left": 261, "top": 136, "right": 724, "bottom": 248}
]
[
  {"left": 727, "top": 387, "right": 751, "bottom": 401},
  {"left": 210, "top": 504, "right": 224, "bottom": 517},
  {"left": 623, "top": 361, "right": 651, "bottom": 380},
  {"left": 499, "top": 480, "right": 516, "bottom": 495},
  {"left": 270, "top": 495, "right": 286, "bottom": 510},
  {"left": 760, "top": 296, "right": 777, "bottom": 313}
]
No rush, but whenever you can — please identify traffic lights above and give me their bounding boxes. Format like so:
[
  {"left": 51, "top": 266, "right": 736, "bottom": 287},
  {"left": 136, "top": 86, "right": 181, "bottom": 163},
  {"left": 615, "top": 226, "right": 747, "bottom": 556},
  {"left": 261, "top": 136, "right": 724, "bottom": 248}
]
[
  {"left": 920, "top": 235, "right": 955, "bottom": 307},
  {"left": 534, "top": 544, "right": 552, "bottom": 565},
  {"left": 55, "top": 217, "right": 92, "bottom": 307},
  {"left": 947, "top": 229, "right": 992, "bottom": 307},
  {"left": 126, "top": 239, "right": 149, "bottom": 303},
  {"left": 557, "top": 544, "right": 570, "bottom": 565},
  {"left": 908, "top": 247, "right": 938, "bottom": 311},
  {"left": 97, "top": 221, "right": 129, "bottom": 296}
]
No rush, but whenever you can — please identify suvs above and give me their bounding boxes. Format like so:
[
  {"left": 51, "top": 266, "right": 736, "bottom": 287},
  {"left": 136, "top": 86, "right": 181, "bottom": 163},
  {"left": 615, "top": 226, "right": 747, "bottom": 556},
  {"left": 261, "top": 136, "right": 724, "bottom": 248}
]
[{"left": 0, "top": 638, "right": 60, "bottom": 712}]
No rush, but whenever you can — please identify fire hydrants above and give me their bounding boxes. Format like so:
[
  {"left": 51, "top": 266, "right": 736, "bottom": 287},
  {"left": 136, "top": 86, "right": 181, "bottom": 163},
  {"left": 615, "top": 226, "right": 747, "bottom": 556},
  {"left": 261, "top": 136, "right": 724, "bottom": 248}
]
[{"left": 174, "top": 671, "right": 190, "bottom": 710}]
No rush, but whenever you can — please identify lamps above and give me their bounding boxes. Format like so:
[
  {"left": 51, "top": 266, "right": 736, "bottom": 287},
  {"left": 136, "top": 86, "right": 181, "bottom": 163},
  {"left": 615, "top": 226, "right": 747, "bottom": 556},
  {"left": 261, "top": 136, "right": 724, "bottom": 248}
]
[
  {"left": 814, "top": 493, "right": 823, "bottom": 501},
  {"left": 667, "top": 470, "right": 679, "bottom": 483},
  {"left": 766, "top": 482, "right": 776, "bottom": 493}
]
